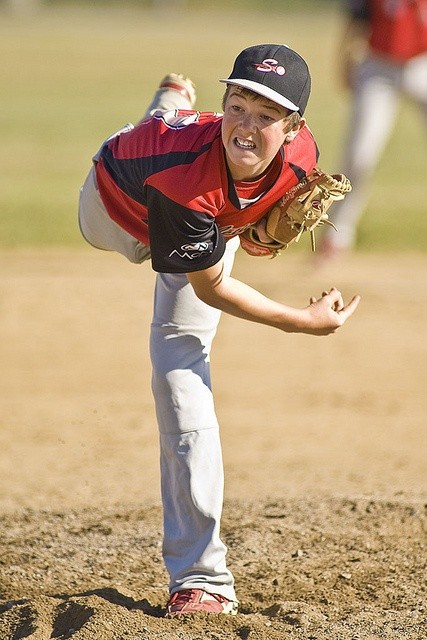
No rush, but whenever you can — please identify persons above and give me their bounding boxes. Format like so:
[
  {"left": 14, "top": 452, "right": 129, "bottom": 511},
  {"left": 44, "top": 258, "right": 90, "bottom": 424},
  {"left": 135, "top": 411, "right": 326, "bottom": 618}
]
[
  {"left": 77, "top": 43, "right": 361, "bottom": 615},
  {"left": 319, "top": 1, "right": 426, "bottom": 253}
]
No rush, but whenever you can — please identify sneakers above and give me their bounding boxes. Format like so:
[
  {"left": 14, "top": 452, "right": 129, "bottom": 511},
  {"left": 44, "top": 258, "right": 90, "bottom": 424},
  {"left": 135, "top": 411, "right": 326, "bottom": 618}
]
[
  {"left": 165, "top": 584, "right": 238, "bottom": 617},
  {"left": 157, "top": 72, "right": 197, "bottom": 107}
]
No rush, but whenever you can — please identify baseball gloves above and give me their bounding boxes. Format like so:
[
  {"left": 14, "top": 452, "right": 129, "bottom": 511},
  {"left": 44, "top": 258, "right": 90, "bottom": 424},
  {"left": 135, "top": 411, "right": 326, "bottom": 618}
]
[{"left": 263, "top": 171, "right": 353, "bottom": 252}]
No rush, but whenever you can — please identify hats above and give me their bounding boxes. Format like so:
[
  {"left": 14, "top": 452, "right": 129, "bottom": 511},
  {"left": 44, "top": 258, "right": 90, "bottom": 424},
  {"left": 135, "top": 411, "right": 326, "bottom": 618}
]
[{"left": 220, "top": 43, "right": 313, "bottom": 118}]
